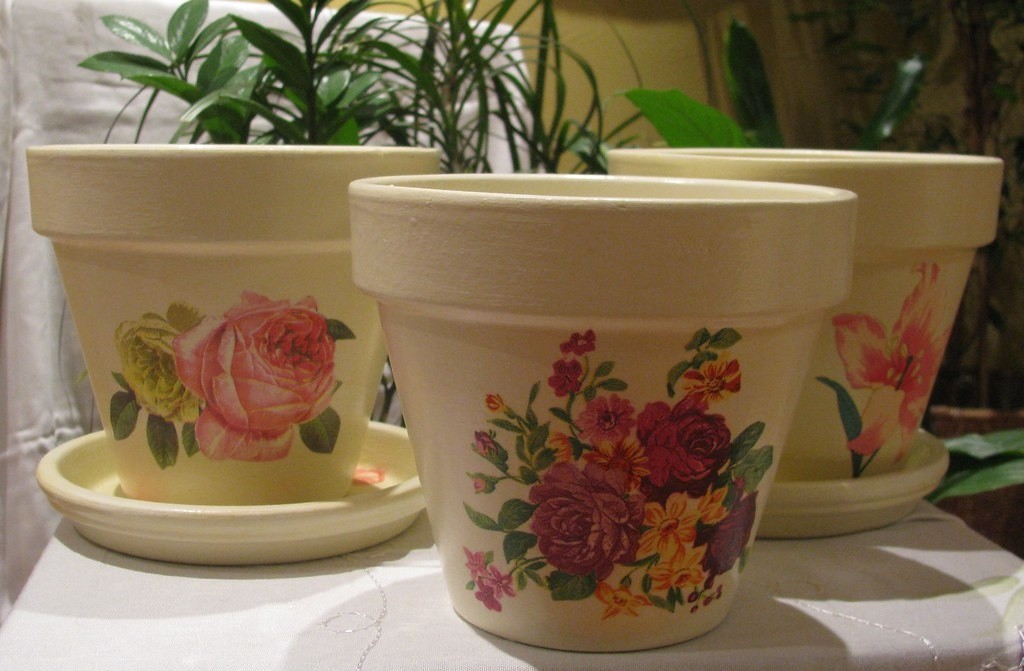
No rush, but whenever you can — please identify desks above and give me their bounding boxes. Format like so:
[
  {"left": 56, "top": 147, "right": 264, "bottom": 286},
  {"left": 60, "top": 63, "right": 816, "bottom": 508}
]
[{"left": 0, "top": 498, "right": 1024, "bottom": 671}]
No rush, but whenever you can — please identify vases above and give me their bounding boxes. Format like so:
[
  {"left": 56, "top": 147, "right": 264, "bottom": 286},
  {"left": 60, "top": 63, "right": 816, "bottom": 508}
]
[
  {"left": 607, "top": 146, "right": 1006, "bottom": 541},
  {"left": 347, "top": 173, "right": 861, "bottom": 654},
  {"left": 22, "top": 143, "right": 443, "bottom": 564}
]
